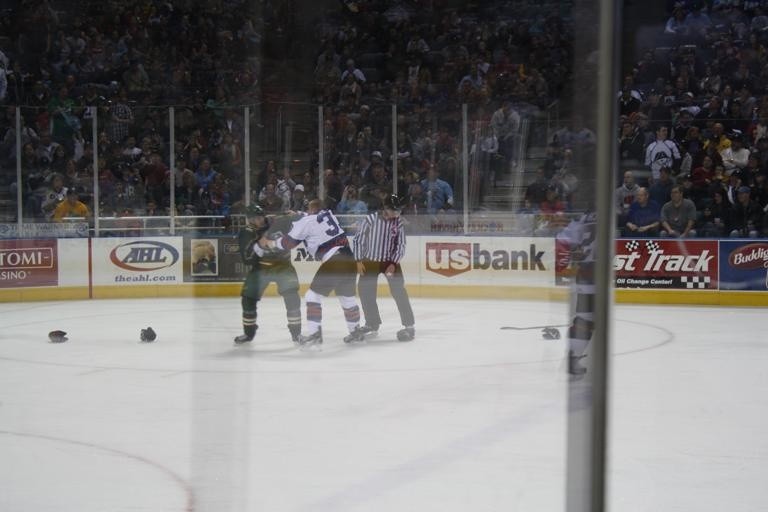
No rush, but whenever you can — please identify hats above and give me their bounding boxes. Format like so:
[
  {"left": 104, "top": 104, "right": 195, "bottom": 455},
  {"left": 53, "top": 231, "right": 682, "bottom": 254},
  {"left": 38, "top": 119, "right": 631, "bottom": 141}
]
[
  {"left": 294, "top": 184, "right": 305, "bottom": 192},
  {"left": 737, "top": 186, "right": 751, "bottom": 193}
]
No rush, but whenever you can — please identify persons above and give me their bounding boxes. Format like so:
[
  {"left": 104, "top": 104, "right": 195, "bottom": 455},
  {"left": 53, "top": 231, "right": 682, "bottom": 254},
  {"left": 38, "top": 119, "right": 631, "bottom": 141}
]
[
  {"left": 345, "top": 192, "right": 416, "bottom": 340},
  {"left": 233, "top": 201, "right": 311, "bottom": 345},
  {"left": 614, "top": 0, "right": 768, "bottom": 239},
  {"left": 255, "top": 197, "right": 364, "bottom": 344},
  {"left": 552, "top": 190, "right": 606, "bottom": 378},
  {"left": 0, "top": 1, "right": 603, "bottom": 225}
]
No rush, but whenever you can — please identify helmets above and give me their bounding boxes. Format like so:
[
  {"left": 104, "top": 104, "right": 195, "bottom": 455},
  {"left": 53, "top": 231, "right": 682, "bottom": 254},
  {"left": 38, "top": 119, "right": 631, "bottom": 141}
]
[
  {"left": 246, "top": 203, "right": 265, "bottom": 217},
  {"left": 384, "top": 193, "right": 402, "bottom": 209}
]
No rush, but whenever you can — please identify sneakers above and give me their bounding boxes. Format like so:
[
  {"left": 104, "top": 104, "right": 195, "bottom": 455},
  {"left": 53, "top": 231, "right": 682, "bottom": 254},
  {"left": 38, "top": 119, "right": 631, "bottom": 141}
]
[
  {"left": 234, "top": 333, "right": 253, "bottom": 344},
  {"left": 292, "top": 333, "right": 323, "bottom": 345},
  {"left": 359, "top": 323, "right": 379, "bottom": 333},
  {"left": 568, "top": 357, "right": 587, "bottom": 375},
  {"left": 344, "top": 332, "right": 365, "bottom": 342},
  {"left": 397, "top": 327, "right": 415, "bottom": 338}
]
